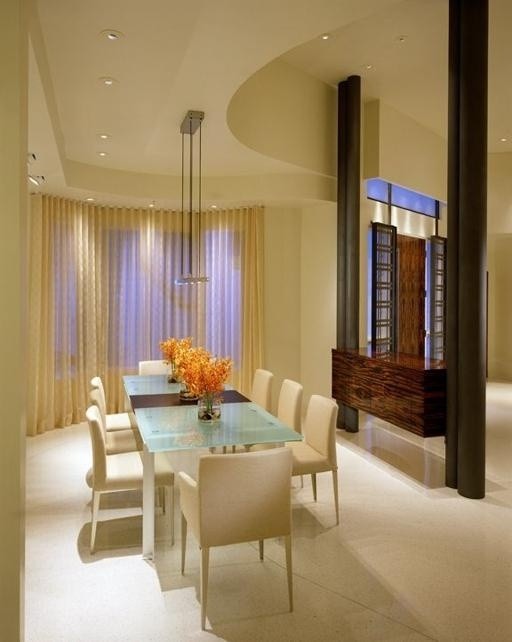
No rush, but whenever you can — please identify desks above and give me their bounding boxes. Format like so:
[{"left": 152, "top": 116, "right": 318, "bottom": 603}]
[{"left": 122, "top": 375, "right": 302, "bottom": 563}]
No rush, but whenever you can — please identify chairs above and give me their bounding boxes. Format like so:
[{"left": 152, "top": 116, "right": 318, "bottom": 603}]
[
  {"left": 251, "top": 396, "right": 340, "bottom": 527},
  {"left": 91, "top": 376, "right": 131, "bottom": 431},
  {"left": 139, "top": 359, "right": 172, "bottom": 377},
  {"left": 211, "top": 369, "right": 274, "bottom": 454},
  {"left": 91, "top": 388, "right": 138, "bottom": 455},
  {"left": 231, "top": 380, "right": 302, "bottom": 454},
  {"left": 86, "top": 405, "right": 175, "bottom": 555},
  {"left": 176, "top": 448, "right": 294, "bottom": 630}
]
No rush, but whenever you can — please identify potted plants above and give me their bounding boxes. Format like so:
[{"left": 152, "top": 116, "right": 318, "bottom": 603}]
[
  {"left": 173, "top": 347, "right": 213, "bottom": 405},
  {"left": 183, "top": 357, "right": 234, "bottom": 423},
  {"left": 160, "top": 337, "right": 192, "bottom": 383}
]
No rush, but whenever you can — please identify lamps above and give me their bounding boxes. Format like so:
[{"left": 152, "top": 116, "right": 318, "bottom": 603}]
[{"left": 175, "top": 110, "right": 209, "bottom": 284}]
